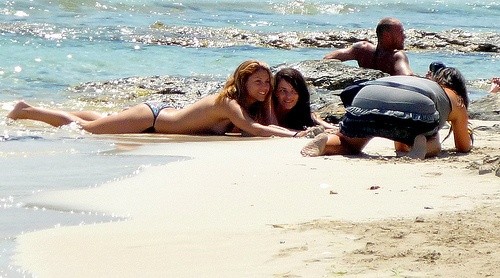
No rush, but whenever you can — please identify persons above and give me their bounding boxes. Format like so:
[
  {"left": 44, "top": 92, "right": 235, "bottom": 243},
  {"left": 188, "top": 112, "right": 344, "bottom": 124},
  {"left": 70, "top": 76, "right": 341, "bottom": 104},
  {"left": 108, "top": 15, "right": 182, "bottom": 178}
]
[
  {"left": 300, "top": 61, "right": 473, "bottom": 161},
  {"left": 6, "top": 59, "right": 307, "bottom": 137},
  {"left": 324, "top": 17, "right": 413, "bottom": 76},
  {"left": 227, "top": 68, "right": 338, "bottom": 133}
]
